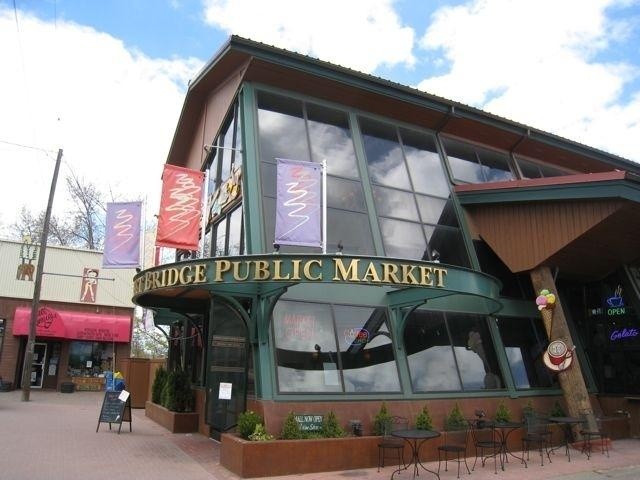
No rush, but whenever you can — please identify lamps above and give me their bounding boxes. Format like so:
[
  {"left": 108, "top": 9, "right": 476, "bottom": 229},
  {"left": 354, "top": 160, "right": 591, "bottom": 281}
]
[
  {"left": 336, "top": 240, "right": 343, "bottom": 255},
  {"left": 431, "top": 249, "right": 441, "bottom": 263},
  {"left": 273, "top": 244, "right": 279, "bottom": 254},
  {"left": 136, "top": 266, "right": 142, "bottom": 273}
]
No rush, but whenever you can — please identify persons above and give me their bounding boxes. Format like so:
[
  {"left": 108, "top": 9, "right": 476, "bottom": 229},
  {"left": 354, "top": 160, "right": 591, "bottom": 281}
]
[{"left": 81, "top": 269, "right": 98, "bottom": 302}]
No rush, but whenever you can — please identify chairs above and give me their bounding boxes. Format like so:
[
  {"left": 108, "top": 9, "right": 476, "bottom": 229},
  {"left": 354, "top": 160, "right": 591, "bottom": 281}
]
[{"left": 377, "top": 407, "right": 609, "bottom": 480}]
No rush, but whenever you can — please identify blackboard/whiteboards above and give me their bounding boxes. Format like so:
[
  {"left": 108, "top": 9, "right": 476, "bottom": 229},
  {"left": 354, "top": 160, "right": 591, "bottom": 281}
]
[{"left": 99, "top": 391, "right": 127, "bottom": 423}]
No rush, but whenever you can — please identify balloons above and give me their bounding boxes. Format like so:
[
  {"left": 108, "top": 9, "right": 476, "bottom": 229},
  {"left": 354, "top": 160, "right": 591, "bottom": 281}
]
[{"left": 536, "top": 289, "right": 556, "bottom": 305}]
